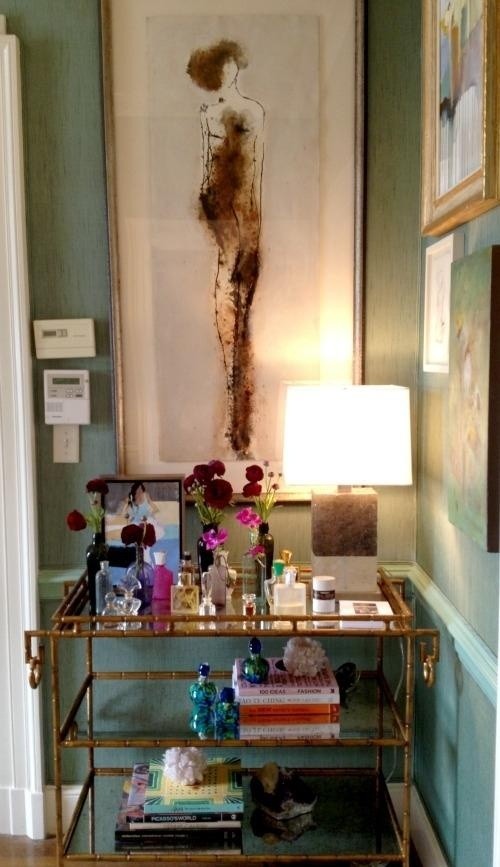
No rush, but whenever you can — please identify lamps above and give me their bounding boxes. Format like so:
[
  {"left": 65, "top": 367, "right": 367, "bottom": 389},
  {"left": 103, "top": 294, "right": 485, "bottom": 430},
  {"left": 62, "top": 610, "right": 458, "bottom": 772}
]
[{"left": 280, "top": 379, "right": 417, "bottom": 594}]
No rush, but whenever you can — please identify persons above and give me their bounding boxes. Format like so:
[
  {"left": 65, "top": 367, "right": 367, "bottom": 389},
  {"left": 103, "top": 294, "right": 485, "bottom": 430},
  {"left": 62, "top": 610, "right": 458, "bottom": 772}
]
[{"left": 122, "top": 484, "right": 165, "bottom": 546}]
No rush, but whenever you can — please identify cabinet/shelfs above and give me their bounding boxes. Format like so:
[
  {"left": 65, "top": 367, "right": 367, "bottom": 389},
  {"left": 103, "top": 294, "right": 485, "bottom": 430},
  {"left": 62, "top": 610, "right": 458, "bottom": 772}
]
[{"left": 23, "top": 564, "right": 439, "bottom": 867}]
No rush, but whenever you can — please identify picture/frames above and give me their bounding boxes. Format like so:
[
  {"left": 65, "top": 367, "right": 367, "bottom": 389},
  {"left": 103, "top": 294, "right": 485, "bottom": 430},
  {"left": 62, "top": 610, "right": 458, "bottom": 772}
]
[
  {"left": 419, "top": 0, "right": 500, "bottom": 238},
  {"left": 423, "top": 234, "right": 452, "bottom": 377},
  {"left": 97, "top": 1, "right": 362, "bottom": 483}
]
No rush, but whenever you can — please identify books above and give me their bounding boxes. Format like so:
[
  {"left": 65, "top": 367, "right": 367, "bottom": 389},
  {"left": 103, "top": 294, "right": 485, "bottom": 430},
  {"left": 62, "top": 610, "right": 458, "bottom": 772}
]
[
  {"left": 114, "top": 756, "right": 245, "bottom": 855},
  {"left": 232, "top": 657, "right": 341, "bottom": 740}
]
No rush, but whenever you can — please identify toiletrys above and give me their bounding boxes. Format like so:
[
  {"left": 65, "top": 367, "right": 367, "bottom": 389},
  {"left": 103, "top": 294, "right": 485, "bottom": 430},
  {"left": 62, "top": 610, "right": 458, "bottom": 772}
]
[{"left": 92, "top": 543, "right": 338, "bottom": 631}]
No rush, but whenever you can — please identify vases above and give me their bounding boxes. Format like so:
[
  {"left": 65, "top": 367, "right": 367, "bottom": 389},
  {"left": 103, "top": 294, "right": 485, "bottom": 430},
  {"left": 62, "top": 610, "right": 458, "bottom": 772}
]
[
  {"left": 85, "top": 532, "right": 107, "bottom": 620},
  {"left": 123, "top": 562, "right": 153, "bottom": 617},
  {"left": 254, "top": 521, "right": 275, "bottom": 565},
  {"left": 242, "top": 552, "right": 267, "bottom": 602}
]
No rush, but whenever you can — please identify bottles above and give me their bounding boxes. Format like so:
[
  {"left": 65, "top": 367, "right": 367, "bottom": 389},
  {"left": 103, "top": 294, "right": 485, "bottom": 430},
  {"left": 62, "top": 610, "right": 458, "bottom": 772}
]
[
  {"left": 188, "top": 664, "right": 214, "bottom": 734},
  {"left": 240, "top": 637, "right": 269, "bottom": 684},
  {"left": 86, "top": 522, "right": 335, "bottom": 632},
  {"left": 216, "top": 687, "right": 239, "bottom": 740}
]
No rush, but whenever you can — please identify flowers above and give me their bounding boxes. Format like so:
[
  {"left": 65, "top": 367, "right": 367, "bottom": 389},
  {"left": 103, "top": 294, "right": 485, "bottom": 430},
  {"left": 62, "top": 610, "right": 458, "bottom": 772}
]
[
  {"left": 233, "top": 506, "right": 262, "bottom": 561},
  {"left": 237, "top": 460, "right": 287, "bottom": 525},
  {"left": 180, "top": 456, "right": 235, "bottom": 526},
  {"left": 67, "top": 477, "right": 110, "bottom": 535},
  {"left": 199, "top": 524, "right": 242, "bottom": 592},
  {"left": 121, "top": 521, "right": 166, "bottom": 553}
]
